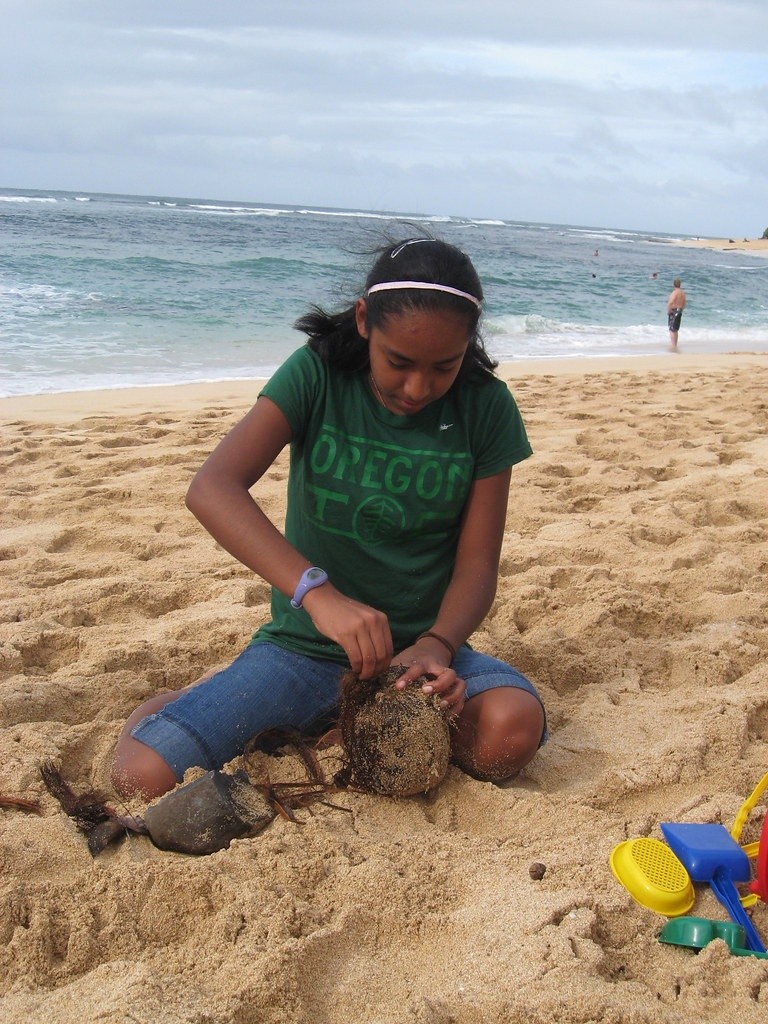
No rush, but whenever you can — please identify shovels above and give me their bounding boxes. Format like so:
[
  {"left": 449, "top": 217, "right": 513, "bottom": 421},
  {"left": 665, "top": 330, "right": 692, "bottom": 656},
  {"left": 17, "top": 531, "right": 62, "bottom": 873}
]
[
  {"left": 658, "top": 915, "right": 767, "bottom": 960},
  {"left": 660, "top": 822, "right": 766, "bottom": 952}
]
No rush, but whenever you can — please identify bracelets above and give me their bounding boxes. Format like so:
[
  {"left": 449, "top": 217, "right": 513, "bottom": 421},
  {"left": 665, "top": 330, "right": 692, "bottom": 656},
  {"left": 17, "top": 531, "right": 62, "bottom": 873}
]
[{"left": 417, "top": 631, "right": 455, "bottom": 668}]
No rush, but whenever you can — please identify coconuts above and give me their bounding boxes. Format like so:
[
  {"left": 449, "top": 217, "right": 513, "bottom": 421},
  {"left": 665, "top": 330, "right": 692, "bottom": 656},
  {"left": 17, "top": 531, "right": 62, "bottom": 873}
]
[{"left": 337, "top": 663, "right": 452, "bottom": 795}]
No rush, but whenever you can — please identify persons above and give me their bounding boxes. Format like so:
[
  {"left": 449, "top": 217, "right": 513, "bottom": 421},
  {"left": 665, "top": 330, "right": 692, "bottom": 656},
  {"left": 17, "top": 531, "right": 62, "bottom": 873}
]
[
  {"left": 594, "top": 250, "right": 600, "bottom": 256},
  {"left": 666, "top": 280, "right": 686, "bottom": 352},
  {"left": 111, "top": 237, "right": 545, "bottom": 803},
  {"left": 650, "top": 273, "right": 657, "bottom": 278},
  {"left": 592, "top": 273, "right": 596, "bottom": 278}
]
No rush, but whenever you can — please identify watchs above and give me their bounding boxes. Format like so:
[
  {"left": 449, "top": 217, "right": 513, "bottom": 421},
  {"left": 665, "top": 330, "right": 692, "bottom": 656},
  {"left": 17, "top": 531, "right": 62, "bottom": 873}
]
[{"left": 290, "top": 567, "right": 328, "bottom": 609}]
